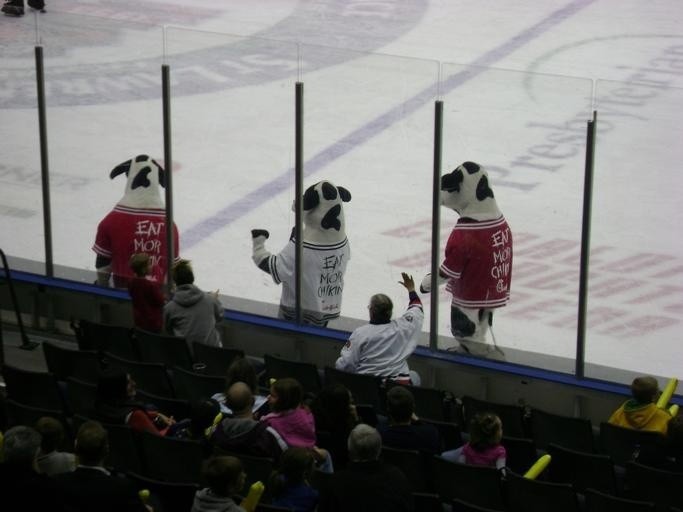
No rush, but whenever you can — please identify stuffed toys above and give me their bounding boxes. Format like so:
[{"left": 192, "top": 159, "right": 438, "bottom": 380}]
[
  {"left": 420, "top": 160, "right": 515, "bottom": 359},
  {"left": 251, "top": 179, "right": 350, "bottom": 326},
  {"left": 91, "top": 152, "right": 180, "bottom": 288}
]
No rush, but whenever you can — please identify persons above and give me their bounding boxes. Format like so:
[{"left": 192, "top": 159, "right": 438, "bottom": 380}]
[
  {"left": 128, "top": 252, "right": 166, "bottom": 334},
  {"left": 0, "top": 354, "right": 509, "bottom": 509},
  {"left": 163, "top": 258, "right": 225, "bottom": 349},
  {"left": 335, "top": 270, "right": 423, "bottom": 386},
  {"left": 607, "top": 375, "right": 678, "bottom": 433}
]
